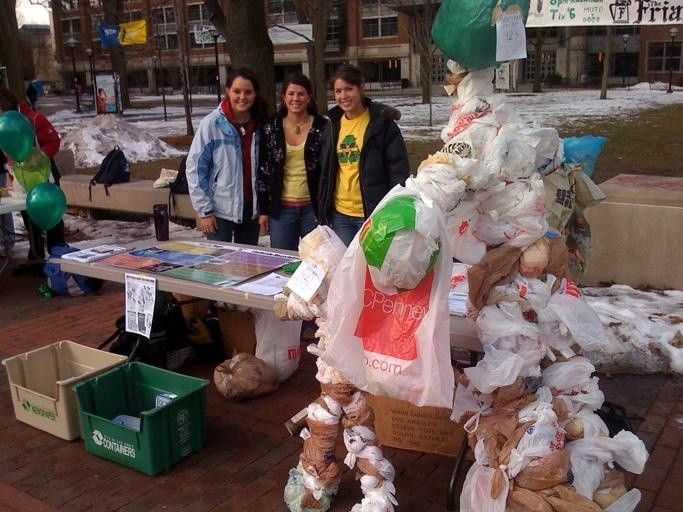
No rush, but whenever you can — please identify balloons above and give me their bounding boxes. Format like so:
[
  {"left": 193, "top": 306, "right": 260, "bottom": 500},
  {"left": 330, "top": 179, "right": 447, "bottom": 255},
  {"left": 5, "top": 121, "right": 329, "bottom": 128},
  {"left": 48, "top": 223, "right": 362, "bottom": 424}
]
[
  {"left": 0, "top": 111, "right": 36, "bottom": 164},
  {"left": 8, "top": 146, "right": 55, "bottom": 187},
  {"left": 24, "top": 181, "right": 68, "bottom": 235}
]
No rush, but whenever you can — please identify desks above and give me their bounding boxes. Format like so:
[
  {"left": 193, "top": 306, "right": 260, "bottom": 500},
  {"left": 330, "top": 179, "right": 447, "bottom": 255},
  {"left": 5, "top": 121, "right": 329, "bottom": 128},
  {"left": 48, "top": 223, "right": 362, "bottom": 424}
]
[{"left": 60, "top": 235, "right": 488, "bottom": 362}]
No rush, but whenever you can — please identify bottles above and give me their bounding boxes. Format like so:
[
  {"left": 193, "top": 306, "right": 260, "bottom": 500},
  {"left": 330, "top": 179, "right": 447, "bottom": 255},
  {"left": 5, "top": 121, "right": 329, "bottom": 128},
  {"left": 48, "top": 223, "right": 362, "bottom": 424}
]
[{"left": 154, "top": 204, "right": 169, "bottom": 240}]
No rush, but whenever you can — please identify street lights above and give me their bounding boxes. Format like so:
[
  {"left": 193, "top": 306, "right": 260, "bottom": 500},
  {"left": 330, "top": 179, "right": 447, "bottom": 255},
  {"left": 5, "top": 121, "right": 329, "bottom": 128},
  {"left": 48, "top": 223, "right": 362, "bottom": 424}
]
[
  {"left": 622, "top": 33, "right": 629, "bottom": 86},
  {"left": 85, "top": 47, "right": 93, "bottom": 106},
  {"left": 666, "top": 28, "right": 680, "bottom": 93},
  {"left": 208, "top": 28, "right": 221, "bottom": 106},
  {"left": 66, "top": 37, "right": 80, "bottom": 112},
  {"left": 152, "top": 56, "right": 158, "bottom": 96}
]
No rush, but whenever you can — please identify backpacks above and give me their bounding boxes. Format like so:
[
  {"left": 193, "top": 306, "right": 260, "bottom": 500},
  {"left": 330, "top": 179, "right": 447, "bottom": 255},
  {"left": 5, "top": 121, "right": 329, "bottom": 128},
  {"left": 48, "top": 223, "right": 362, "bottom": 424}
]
[
  {"left": 90, "top": 144, "right": 131, "bottom": 186},
  {"left": 169, "top": 155, "right": 189, "bottom": 194}
]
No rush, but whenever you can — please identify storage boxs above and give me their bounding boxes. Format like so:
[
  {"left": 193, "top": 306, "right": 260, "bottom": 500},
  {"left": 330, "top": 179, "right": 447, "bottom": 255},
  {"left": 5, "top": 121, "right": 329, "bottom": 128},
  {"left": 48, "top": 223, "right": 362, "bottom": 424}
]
[
  {"left": 69, "top": 362, "right": 211, "bottom": 476},
  {"left": 215, "top": 307, "right": 258, "bottom": 361},
  {"left": 371, "top": 374, "right": 477, "bottom": 462},
  {"left": 170, "top": 291, "right": 210, "bottom": 322},
  {"left": 2, "top": 340, "right": 128, "bottom": 442}
]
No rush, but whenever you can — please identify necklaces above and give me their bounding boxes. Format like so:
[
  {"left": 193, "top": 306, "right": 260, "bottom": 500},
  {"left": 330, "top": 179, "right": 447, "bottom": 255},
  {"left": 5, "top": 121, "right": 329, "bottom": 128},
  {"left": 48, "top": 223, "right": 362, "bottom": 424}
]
[
  {"left": 285, "top": 116, "right": 308, "bottom": 135},
  {"left": 233, "top": 114, "right": 246, "bottom": 136}
]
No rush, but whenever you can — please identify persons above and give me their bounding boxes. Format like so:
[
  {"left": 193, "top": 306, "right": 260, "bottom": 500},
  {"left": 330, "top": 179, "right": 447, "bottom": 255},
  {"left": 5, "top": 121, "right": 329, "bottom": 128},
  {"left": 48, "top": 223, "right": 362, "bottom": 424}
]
[
  {"left": 25, "top": 82, "right": 39, "bottom": 112},
  {"left": 252, "top": 71, "right": 337, "bottom": 253},
  {"left": 184, "top": 65, "right": 262, "bottom": 247},
  {"left": 0, "top": 80, "right": 68, "bottom": 273},
  {"left": 320, "top": 63, "right": 411, "bottom": 248},
  {"left": 95, "top": 88, "right": 107, "bottom": 114}
]
